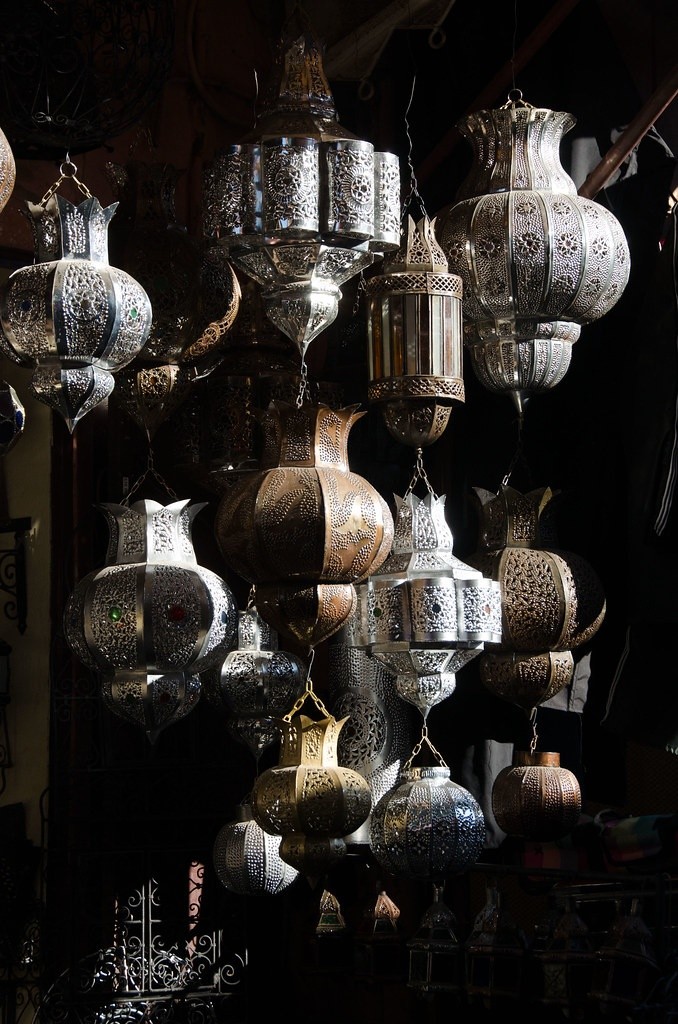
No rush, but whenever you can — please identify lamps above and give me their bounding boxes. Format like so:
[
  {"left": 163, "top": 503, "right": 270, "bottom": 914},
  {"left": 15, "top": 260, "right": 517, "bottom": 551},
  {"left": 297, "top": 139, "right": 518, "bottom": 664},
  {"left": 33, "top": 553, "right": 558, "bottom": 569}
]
[{"left": 0, "top": 33, "right": 663, "bottom": 1024}]
